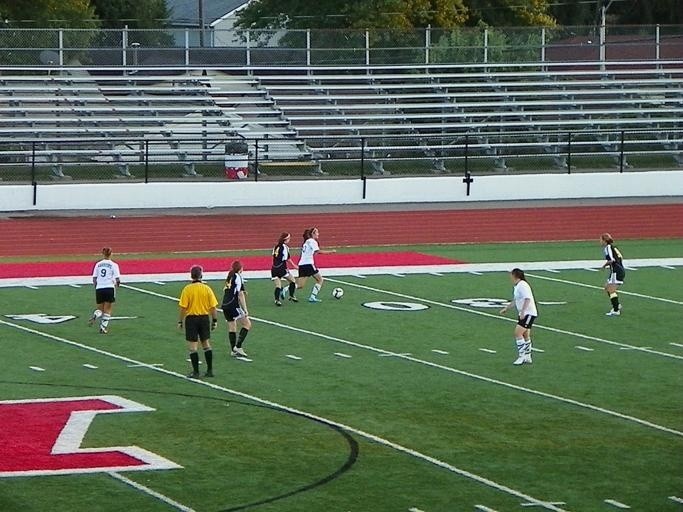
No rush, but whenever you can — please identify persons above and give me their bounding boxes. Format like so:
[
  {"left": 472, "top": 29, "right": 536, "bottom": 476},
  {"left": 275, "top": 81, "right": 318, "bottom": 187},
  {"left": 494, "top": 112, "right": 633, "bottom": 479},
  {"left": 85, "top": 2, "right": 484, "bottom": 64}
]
[
  {"left": 88, "top": 247, "right": 120, "bottom": 333},
  {"left": 281, "top": 226, "right": 336, "bottom": 302},
  {"left": 500, "top": 268, "right": 538, "bottom": 365},
  {"left": 271, "top": 231, "right": 299, "bottom": 306},
  {"left": 177, "top": 265, "right": 218, "bottom": 378},
  {"left": 600, "top": 233, "right": 626, "bottom": 316},
  {"left": 220, "top": 260, "right": 251, "bottom": 356}
]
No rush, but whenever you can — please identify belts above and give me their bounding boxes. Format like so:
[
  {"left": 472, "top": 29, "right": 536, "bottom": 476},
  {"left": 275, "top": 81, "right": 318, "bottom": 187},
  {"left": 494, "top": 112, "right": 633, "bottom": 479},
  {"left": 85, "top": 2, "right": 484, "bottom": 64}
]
[{"left": 186, "top": 314, "right": 208, "bottom": 318}]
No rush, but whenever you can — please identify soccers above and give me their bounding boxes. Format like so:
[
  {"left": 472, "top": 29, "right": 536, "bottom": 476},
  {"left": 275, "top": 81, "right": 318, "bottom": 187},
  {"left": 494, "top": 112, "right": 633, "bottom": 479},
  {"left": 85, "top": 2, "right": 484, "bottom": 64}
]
[{"left": 333, "top": 288, "right": 344, "bottom": 299}]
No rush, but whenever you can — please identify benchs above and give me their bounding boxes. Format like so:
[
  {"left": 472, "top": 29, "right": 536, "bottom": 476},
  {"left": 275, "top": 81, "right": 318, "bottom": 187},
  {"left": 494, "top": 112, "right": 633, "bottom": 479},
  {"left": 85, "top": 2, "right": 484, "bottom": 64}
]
[{"left": 0, "top": 61, "right": 683, "bottom": 176}]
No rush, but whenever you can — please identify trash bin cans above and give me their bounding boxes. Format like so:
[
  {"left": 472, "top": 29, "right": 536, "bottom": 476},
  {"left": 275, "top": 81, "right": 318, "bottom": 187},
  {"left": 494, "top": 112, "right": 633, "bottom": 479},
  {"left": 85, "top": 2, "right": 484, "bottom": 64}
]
[{"left": 225, "top": 142, "right": 248, "bottom": 178}]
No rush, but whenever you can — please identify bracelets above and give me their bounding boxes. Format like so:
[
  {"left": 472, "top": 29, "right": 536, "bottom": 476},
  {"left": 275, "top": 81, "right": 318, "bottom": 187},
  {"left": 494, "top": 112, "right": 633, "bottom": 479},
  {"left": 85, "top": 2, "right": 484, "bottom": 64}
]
[
  {"left": 212, "top": 318, "right": 217, "bottom": 322},
  {"left": 178, "top": 322, "right": 182, "bottom": 324}
]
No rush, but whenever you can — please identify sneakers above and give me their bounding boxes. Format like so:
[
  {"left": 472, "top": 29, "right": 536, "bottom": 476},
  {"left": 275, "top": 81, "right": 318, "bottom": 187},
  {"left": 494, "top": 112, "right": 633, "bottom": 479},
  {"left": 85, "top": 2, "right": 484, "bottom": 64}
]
[
  {"left": 513, "top": 356, "right": 525, "bottom": 365},
  {"left": 231, "top": 352, "right": 242, "bottom": 357},
  {"left": 186, "top": 372, "right": 199, "bottom": 379},
  {"left": 281, "top": 287, "right": 286, "bottom": 300},
  {"left": 610, "top": 304, "right": 622, "bottom": 312},
  {"left": 233, "top": 345, "right": 248, "bottom": 357},
  {"left": 308, "top": 297, "right": 322, "bottom": 303},
  {"left": 605, "top": 310, "right": 621, "bottom": 316},
  {"left": 274, "top": 299, "right": 282, "bottom": 306},
  {"left": 526, "top": 358, "right": 533, "bottom": 364},
  {"left": 99, "top": 325, "right": 107, "bottom": 334},
  {"left": 288, "top": 295, "right": 298, "bottom": 302},
  {"left": 87, "top": 314, "right": 97, "bottom": 327}
]
